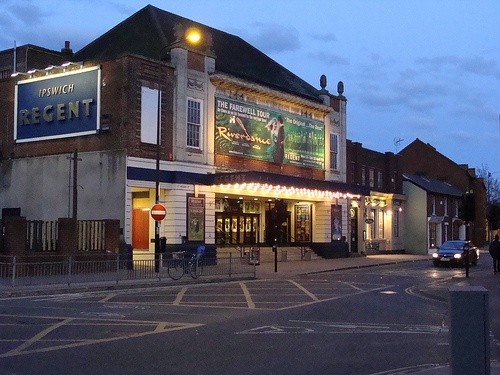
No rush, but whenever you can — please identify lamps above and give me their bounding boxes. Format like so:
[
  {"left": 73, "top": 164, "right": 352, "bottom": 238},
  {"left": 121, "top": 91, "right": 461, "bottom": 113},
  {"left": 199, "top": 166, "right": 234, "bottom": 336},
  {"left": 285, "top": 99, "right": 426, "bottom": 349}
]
[{"left": 11, "top": 61, "right": 83, "bottom": 79}]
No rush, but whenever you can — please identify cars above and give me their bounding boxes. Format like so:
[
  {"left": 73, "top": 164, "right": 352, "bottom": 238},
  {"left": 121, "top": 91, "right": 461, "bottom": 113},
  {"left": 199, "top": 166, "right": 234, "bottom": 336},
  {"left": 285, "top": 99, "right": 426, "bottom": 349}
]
[{"left": 432, "top": 240, "right": 480, "bottom": 268}]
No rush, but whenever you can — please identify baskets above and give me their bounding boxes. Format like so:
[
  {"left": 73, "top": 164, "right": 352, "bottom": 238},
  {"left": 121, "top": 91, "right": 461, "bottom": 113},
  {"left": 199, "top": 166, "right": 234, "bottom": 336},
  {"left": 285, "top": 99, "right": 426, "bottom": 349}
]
[{"left": 172, "top": 253, "right": 183, "bottom": 259}]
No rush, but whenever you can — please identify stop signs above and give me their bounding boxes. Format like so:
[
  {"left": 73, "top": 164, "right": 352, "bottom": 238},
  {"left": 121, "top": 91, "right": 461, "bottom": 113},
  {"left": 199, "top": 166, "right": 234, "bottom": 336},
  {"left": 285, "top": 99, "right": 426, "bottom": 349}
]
[{"left": 151, "top": 204, "right": 167, "bottom": 222}]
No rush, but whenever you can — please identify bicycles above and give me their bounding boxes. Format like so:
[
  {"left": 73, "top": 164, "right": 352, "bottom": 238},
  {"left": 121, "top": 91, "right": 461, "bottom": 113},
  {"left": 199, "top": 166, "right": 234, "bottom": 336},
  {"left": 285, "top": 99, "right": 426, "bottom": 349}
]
[{"left": 167, "top": 250, "right": 204, "bottom": 280}]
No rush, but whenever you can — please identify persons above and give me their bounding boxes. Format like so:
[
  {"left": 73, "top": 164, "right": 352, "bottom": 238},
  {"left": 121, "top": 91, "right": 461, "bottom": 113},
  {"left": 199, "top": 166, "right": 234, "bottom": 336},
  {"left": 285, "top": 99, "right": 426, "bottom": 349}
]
[
  {"left": 176, "top": 236, "right": 194, "bottom": 273},
  {"left": 265, "top": 116, "right": 285, "bottom": 164},
  {"left": 489, "top": 234, "right": 500, "bottom": 274}
]
